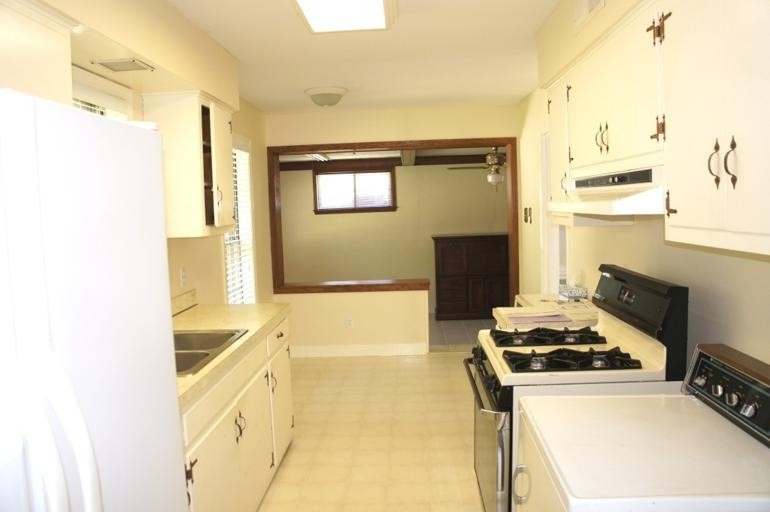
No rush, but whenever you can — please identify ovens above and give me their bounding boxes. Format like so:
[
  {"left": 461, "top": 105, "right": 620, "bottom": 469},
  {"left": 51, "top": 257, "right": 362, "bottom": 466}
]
[{"left": 462, "top": 344, "right": 512, "bottom": 512}]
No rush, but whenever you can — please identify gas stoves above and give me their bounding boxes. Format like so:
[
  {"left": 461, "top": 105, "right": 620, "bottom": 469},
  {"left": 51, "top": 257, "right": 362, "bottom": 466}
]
[{"left": 477, "top": 308, "right": 668, "bottom": 386}]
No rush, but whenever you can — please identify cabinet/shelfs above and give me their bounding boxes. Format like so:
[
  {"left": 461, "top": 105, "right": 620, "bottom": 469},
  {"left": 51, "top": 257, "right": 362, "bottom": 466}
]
[
  {"left": 133, "top": 88, "right": 234, "bottom": 238},
  {"left": 662, "top": 1, "right": 770, "bottom": 234},
  {"left": 562, "top": 0, "right": 662, "bottom": 170},
  {"left": 430, "top": 235, "right": 508, "bottom": 320},
  {"left": 181, "top": 340, "right": 280, "bottom": 512},
  {"left": 267, "top": 316, "right": 295, "bottom": 468}
]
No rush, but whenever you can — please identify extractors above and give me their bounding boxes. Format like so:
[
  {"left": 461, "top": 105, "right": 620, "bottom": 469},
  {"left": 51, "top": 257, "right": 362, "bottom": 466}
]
[{"left": 570, "top": 164, "right": 668, "bottom": 215}]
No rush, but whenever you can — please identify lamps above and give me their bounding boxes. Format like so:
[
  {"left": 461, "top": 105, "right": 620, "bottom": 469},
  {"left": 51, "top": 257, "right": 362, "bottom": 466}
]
[
  {"left": 302, "top": 86, "right": 349, "bottom": 107},
  {"left": 486, "top": 169, "right": 505, "bottom": 192}
]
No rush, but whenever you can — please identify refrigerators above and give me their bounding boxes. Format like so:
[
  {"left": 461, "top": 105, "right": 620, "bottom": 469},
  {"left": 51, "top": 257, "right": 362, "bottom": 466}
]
[{"left": 0, "top": 88, "right": 190, "bottom": 511}]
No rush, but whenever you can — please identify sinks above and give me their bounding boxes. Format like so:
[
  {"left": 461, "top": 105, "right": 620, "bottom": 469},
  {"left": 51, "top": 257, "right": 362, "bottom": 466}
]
[
  {"left": 173, "top": 329, "right": 246, "bottom": 352},
  {"left": 175, "top": 351, "right": 211, "bottom": 374}
]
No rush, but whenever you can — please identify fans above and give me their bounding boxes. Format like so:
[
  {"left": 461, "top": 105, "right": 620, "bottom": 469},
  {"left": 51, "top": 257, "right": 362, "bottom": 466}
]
[{"left": 447, "top": 147, "right": 507, "bottom": 169}]
